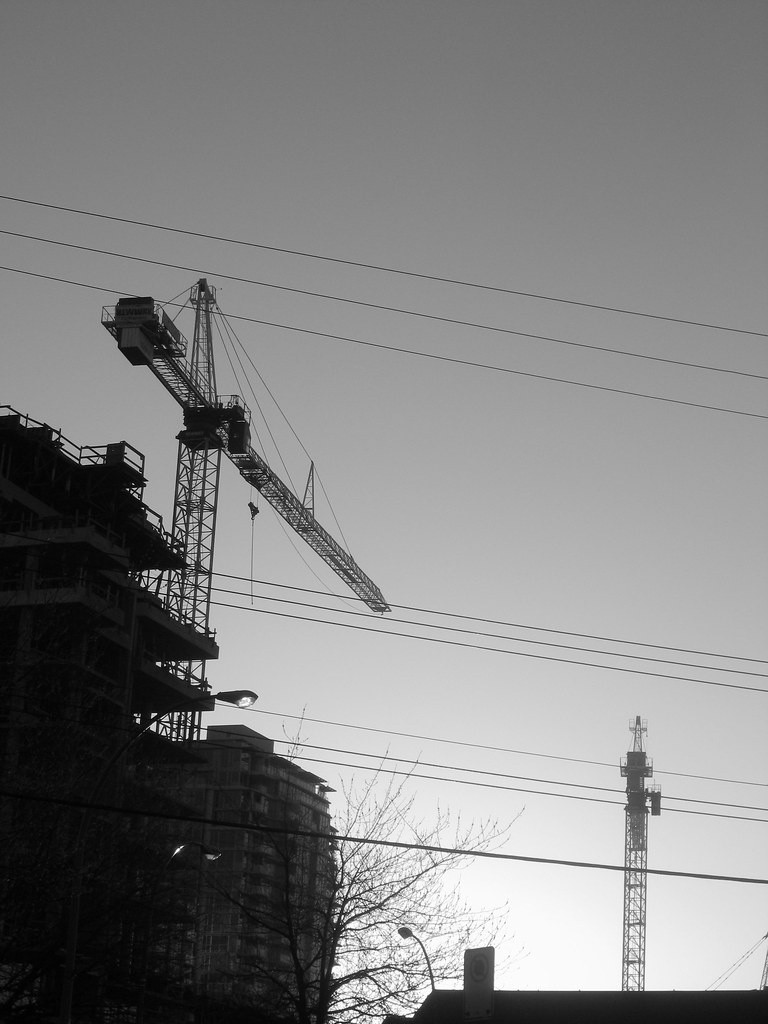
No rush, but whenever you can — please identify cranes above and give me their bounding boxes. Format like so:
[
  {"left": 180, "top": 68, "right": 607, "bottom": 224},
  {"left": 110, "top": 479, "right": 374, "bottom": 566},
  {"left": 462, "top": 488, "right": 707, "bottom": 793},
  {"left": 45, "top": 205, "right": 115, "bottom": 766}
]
[{"left": 100, "top": 276, "right": 393, "bottom": 746}]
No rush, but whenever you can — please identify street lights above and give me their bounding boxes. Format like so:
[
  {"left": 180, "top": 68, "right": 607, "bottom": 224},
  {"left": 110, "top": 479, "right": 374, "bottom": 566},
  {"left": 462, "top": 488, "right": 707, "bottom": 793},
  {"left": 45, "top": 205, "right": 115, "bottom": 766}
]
[{"left": 55, "top": 689, "right": 258, "bottom": 1024}]
